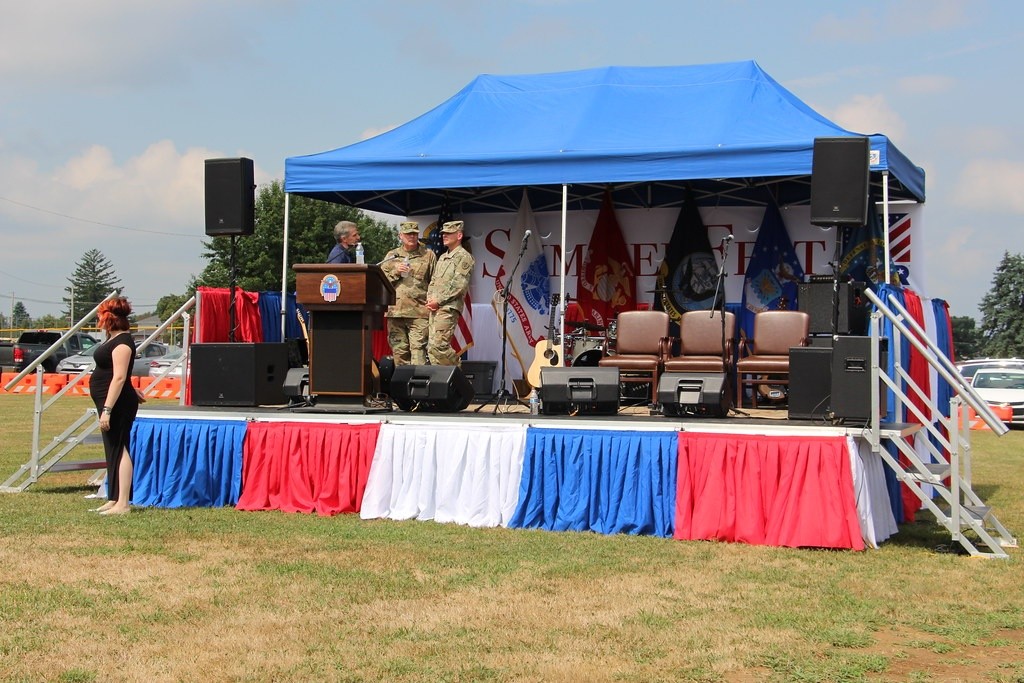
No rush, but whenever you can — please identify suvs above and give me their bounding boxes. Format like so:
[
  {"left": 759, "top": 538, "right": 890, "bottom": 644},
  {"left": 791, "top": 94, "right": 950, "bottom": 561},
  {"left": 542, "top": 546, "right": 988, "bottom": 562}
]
[{"left": 954, "top": 357, "right": 1024, "bottom": 377}]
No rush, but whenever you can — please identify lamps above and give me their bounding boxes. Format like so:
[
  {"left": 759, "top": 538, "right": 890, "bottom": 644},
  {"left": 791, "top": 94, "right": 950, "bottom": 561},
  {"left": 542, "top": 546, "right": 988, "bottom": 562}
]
[
  {"left": 540, "top": 366, "right": 621, "bottom": 417},
  {"left": 391, "top": 365, "right": 475, "bottom": 413},
  {"left": 656, "top": 372, "right": 731, "bottom": 419},
  {"left": 283, "top": 368, "right": 314, "bottom": 407}
]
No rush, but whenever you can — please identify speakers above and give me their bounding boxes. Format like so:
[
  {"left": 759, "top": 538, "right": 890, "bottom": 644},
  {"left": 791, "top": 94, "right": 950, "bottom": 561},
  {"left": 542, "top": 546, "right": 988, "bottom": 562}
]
[
  {"left": 283, "top": 367, "right": 310, "bottom": 404},
  {"left": 618, "top": 374, "right": 653, "bottom": 407},
  {"left": 829, "top": 336, "right": 888, "bottom": 420},
  {"left": 810, "top": 137, "right": 871, "bottom": 228},
  {"left": 657, "top": 372, "right": 733, "bottom": 419},
  {"left": 391, "top": 364, "right": 474, "bottom": 414},
  {"left": 204, "top": 157, "right": 257, "bottom": 236},
  {"left": 796, "top": 281, "right": 867, "bottom": 336},
  {"left": 190, "top": 341, "right": 291, "bottom": 407},
  {"left": 788, "top": 347, "right": 834, "bottom": 420},
  {"left": 540, "top": 366, "right": 620, "bottom": 416}
]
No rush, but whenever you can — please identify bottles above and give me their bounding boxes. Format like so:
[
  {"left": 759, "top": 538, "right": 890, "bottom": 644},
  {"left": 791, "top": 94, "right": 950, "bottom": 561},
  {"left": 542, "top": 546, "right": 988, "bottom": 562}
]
[
  {"left": 530, "top": 390, "right": 539, "bottom": 415},
  {"left": 355, "top": 242, "right": 365, "bottom": 264},
  {"left": 400, "top": 256, "right": 410, "bottom": 278}
]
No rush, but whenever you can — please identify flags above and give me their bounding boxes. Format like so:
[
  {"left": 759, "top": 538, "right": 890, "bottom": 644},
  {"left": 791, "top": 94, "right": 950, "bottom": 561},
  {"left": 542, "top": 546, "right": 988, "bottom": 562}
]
[
  {"left": 430, "top": 196, "right": 475, "bottom": 358},
  {"left": 653, "top": 186, "right": 726, "bottom": 354},
  {"left": 830, "top": 193, "right": 903, "bottom": 316},
  {"left": 494, "top": 184, "right": 552, "bottom": 393},
  {"left": 576, "top": 184, "right": 637, "bottom": 356},
  {"left": 736, "top": 194, "right": 806, "bottom": 354}
]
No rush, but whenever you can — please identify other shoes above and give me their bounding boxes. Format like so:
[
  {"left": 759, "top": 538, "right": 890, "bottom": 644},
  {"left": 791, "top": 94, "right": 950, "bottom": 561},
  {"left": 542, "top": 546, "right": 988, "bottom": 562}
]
[
  {"left": 100, "top": 508, "right": 131, "bottom": 516},
  {"left": 88, "top": 507, "right": 110, "bottom": 512}
]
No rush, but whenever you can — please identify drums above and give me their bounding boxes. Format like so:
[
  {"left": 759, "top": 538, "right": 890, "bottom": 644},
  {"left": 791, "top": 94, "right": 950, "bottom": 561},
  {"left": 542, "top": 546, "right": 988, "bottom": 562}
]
[
  {"left": 609, "top": 321, "right": 617, "bottom": 339},
  {"left": 572, "top": 336, "right": 606, "bottom": 363},
  {"left": 571, "top": 348, "right": 612, "bottom": 368}
]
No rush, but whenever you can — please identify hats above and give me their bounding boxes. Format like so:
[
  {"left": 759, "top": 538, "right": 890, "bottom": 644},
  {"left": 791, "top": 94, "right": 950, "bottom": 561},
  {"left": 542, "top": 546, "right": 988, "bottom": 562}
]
[
  {"left": 439, "top": 221, "right": 464, "bottom": 233},
  {"left": 400, "top": 221, "right": 419, "bottom": 233}
]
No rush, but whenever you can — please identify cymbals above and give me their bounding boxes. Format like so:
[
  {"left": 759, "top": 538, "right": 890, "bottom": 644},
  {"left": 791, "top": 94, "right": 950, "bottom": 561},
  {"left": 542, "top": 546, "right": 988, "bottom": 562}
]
[
  {"left": 644, "top": 289, "right": 681, "bottom": 294},
  {"left": 565, "top": 321, "right": 606, "bottom": 332}
]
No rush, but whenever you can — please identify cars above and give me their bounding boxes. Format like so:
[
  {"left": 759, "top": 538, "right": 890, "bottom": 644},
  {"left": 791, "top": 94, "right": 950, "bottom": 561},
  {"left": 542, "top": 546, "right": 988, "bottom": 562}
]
[
  {"left": 147, "top": 350, "right": 191, "bottom": 377},
  {"left": 55, "top": 339, "right": 170, "bottom": 377},
  {"left": 970, "top": 367, "right": 1024, "bottom": 427}
]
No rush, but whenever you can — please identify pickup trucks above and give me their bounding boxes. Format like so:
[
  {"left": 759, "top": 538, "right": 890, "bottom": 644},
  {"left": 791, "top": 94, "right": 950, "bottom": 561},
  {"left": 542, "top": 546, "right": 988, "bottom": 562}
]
[{"left": 0, "top": 331, "right": 101, "bottom": 374}]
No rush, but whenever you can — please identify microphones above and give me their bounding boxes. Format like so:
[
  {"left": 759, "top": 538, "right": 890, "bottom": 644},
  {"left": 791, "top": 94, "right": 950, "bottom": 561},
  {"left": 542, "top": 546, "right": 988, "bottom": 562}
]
[
  {"left": 348, "top": 242, "right": 363, "bottom": 248},
  {"left": 375, "top": 253, "right": 400, "bottom": 265},
  {"left": 722, "top": 235, "right": 734, "bottom": 241},
  {"left": 522, "top": 230, "right": 532, "bottom": 242}
]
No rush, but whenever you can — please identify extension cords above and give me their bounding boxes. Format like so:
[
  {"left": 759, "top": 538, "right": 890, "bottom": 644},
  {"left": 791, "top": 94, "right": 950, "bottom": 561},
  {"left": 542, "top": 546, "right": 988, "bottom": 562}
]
[{"left": 650, "top": 409, "right": 662, "bottom": 415}]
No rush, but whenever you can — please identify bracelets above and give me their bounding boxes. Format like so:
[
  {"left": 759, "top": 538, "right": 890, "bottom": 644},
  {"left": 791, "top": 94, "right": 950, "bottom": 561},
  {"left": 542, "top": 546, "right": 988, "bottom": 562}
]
[{"left": 102, "top": 407, "right": 112, "bottom": 415}]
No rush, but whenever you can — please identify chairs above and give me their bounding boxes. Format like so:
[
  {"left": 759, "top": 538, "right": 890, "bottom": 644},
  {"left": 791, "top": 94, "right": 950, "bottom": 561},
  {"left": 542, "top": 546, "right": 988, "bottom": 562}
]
[
  {"left": 736, "top": 310, "right": 809, "bottom": 408},
  {"left": 598, "top": 311, "right": 670, "bottom": 417},
  {"left": 663, "top": 310, "right": 735, "bottom": 373}
]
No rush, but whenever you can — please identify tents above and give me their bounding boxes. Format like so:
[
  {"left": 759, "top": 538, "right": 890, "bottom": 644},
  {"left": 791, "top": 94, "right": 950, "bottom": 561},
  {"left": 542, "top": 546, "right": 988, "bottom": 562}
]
[{"left": 280, "top": 61, "right": 927, "bottom": 422}]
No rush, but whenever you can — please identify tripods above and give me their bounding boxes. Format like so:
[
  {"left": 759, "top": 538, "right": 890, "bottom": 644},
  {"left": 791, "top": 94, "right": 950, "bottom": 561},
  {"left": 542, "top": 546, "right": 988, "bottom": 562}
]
[{"left": 474, "top": 238, "right": 531, "bottom": 416}]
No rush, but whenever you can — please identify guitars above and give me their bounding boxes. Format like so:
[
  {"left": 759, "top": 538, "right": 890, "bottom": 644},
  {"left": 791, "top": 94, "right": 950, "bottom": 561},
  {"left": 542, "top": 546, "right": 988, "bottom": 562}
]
[
  {"left": 296, "top": 307, "right": 310, "bottom": 358},
  {"left": 525, "top": 292, "right": 564, "bottom": 389}
]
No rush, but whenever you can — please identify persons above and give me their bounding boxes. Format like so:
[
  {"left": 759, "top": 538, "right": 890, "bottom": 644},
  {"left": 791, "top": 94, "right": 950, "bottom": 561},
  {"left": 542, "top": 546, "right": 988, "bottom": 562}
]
[
  {"left": 89, "top": 298, "right": 147, "bottom": 517},
  {"left": 380, "top": 222, "right": 436, "bottom": 368},
  {"left": 328, "top": 221, "right": 360, "bottom": 264},
  {"left": 423, "top": 221, "right": 475, "bottom": 370}
]
